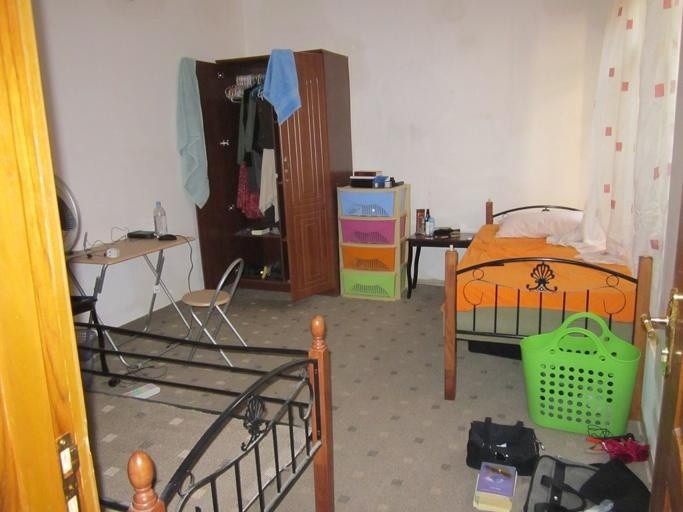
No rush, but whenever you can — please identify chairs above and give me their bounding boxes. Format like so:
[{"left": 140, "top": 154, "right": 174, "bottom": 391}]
[{"left": 181, "top": 257, "right": 248, "bottom": 368}]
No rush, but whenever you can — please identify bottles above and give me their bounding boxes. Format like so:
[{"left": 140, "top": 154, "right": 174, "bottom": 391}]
[
  {"left": 423, "top": 209, "right": 431, "bottom": 237},
  {"left": 153, "top": 201, "right": 166, "bottom": 235}
]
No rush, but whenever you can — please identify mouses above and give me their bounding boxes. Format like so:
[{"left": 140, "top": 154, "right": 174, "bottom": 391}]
[{"left": 158, "top": 234, "right": 177, "bottom": 241}]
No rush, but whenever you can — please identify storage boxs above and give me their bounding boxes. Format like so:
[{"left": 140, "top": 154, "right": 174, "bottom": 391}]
[{"left": 336, "top": 183, "right": 411, "bottom": 302}]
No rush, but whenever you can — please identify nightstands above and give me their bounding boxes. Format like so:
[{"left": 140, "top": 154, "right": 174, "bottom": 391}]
[
  {"left": 70, "top": 296, "right": 110, "bottom": 374},
  {"left": 403, "top": 232, "right": 475, "bottom": 298}
]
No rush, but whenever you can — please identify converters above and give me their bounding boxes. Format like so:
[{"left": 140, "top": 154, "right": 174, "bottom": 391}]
[
  {"left": 123, "top": 383, "right": 161, "bottom": 399},
  {"left": 127, "top": 230, "right": 155, "bottom": 239}
]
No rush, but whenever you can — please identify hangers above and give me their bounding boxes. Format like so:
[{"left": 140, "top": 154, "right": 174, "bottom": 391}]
[{"left": 224, "top": 73, "right": 264, "bottom": 103}]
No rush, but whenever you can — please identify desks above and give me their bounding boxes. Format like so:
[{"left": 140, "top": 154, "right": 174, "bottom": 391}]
[{"left": 69, "top": 235, "right": 196, "bottom": 369}]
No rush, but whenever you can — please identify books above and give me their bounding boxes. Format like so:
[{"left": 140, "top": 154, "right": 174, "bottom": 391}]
[{"left": 349, "top": 170, "right": 383, "bottom": 188}]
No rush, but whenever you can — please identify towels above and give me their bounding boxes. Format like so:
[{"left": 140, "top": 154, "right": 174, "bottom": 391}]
[
  {"left": 263, "top": 49, "right": 303, "bottom": 126},
  {"left": 177, "top": 58, "right": 210, "bottom": 210}
]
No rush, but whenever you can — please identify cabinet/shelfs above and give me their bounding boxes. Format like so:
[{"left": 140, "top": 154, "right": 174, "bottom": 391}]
[{"left": 194, "top": 48, "right": 352, "bottom": 303}]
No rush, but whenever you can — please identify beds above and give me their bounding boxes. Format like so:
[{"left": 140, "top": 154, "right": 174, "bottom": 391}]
[
  {"left": 74, "top": 314, "right": 335, "bottom": 512},
  {"left": 439, "top": 197, "right": 654, "bottom": 421}
]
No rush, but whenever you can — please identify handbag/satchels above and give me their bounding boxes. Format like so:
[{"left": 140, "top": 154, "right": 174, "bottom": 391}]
[
  {"left": 520, "top": 312, "right": 641, "bottom": 438},
  {"left": 523, "top": 455, "right": 599, "bottom": 512},
  {"left": 465, "top": 416, "right": 545, "bottom": 477}
]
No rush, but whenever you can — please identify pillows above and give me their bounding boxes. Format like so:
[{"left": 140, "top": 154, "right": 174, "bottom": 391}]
[{"left": 495, "top": 209, "right": 584, "bottom": 239}]
